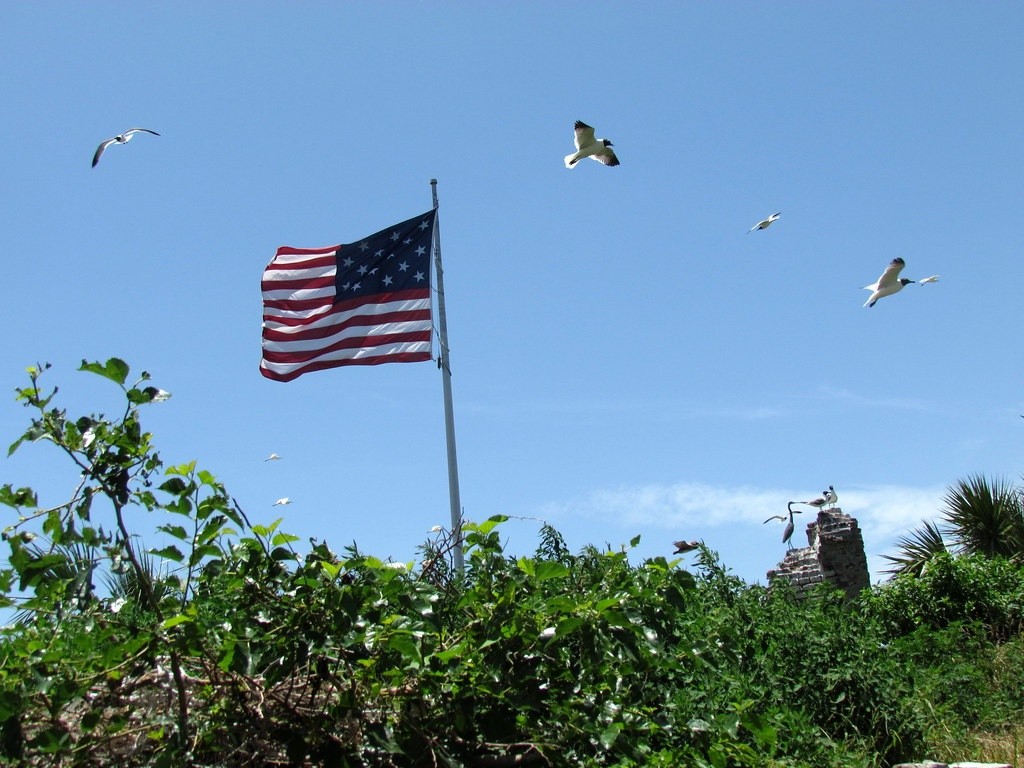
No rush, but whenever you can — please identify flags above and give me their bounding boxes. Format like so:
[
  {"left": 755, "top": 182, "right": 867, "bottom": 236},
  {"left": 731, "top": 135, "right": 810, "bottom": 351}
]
[{"left": 259, "top": 207, "right": 452, "bottom": 382}]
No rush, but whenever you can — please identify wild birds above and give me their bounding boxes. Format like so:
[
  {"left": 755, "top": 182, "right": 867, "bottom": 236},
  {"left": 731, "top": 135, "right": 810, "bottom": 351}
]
[
  {"left": 264, "top": 454, "right": 284, "bottom": 463},
  {"left": 427, "top": 525, "right": 442, "bottom": 533},
  {"left": 564, "top": 120, "right": 621, "bottom": 169},
  {"left": 858, "top": 256, "right": 916, "bottom": 310},
  {"left": 917, "top": 274, "right": 943, "bottom": 288},
  {"left": 91, "top": 128, "right": 161, "bottom": 168},
  {"left": 273, "top": 497, "right": 293, "bottom": 507},
  {"left": 744, "top": 211, "right": 782, "bottom": 235},
  {"left": 763, "top": 485, "right": 838, "bottom": 552}
]
